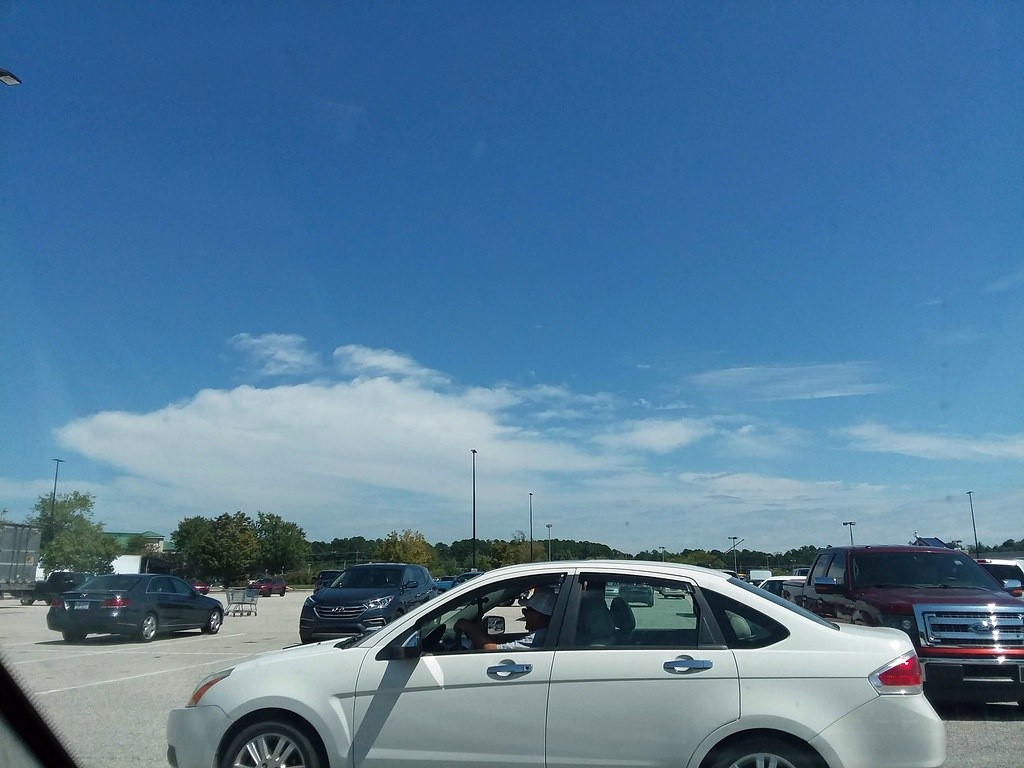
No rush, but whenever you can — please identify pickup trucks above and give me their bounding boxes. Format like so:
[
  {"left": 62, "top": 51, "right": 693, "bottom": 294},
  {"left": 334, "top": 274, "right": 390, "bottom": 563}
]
[{"left": 784, "top": 545, "right": 1024, "bottom": 715}]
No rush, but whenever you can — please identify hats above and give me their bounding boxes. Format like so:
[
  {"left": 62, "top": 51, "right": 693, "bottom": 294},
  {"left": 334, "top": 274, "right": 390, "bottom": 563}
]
[{"left": 518, "top": 592, "right": 559, "bottom": 616}]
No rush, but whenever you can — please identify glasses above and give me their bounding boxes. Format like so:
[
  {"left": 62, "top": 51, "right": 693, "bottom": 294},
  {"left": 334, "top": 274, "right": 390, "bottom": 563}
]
[{"left": 522, "top": 608, "right": 538, "bottom": 616}]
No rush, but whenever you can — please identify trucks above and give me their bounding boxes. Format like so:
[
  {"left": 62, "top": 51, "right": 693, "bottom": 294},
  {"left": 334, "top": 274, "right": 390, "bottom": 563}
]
[{"left": 0, "top": 522, "right": 41, "bottom": 600}]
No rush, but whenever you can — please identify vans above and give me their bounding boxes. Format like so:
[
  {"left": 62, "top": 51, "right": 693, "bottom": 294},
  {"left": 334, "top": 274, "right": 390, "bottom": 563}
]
[{"left": 45, "top": 571, "right": 95, "bottom": 606}]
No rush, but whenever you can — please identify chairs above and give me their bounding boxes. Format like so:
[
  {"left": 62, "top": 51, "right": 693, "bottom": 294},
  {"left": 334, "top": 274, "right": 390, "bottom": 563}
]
[
  {"left": 726, "top": 610, "right": 752, "bottom": 641},
  {"left": 588, "top": 595, "right": 637, "bottom": 647}
]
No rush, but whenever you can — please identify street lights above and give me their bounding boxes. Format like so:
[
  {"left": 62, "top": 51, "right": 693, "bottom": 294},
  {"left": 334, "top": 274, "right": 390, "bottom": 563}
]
[
  {"left": 659, "top": 546, "right": 666, "bottom": 562},
  {"left": 46, "top": 458, "right": 66, "bottom": 546},
  {"left": 843, "top": 521, "right": 856, "bottom": 545},
  {"left": 966, "top": 490, "right": 980, "bottom": 559},
  {"left": 470, "top": 449, "right": 477, "bottom": 568},
  {"left": 728, "top": 536, "right": 738, "bottom": 578},
  {"left": 528, "top": 492, "right": 534, "bottom": 562},
  {"left": 545, "top": 523, "right": 553, "bottom": 561}
]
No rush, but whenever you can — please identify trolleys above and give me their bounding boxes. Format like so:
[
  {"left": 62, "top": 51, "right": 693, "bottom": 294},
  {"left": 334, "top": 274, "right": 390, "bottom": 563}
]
[{"left": 221, "top": 586, "right": 261, "bottom": 617}]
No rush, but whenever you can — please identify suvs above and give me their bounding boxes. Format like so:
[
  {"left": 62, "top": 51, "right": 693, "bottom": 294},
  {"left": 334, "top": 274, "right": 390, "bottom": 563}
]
[
  {"left": 245, "top": 575, "right": 287, "bottom": 598},
  {"left": 299, "top": 562, "right": 439, "bottom": 644}
]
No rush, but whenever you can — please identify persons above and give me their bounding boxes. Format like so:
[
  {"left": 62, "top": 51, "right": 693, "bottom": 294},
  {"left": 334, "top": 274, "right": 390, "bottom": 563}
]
[{"left": 453, "top": 590, "right": 558, "bottom": 652}]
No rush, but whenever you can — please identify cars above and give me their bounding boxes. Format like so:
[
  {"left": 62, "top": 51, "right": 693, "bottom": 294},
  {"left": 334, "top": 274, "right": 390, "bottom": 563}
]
[
  {"left": 756, "top": 575, "right": 809, "bottom": 607},
  {"left": 164, "top": 557, "right": 948, "bottom": 768},
  {"left": 953, "top": 557, "right": 1024, "bottom": 602},
  {"left": 311, "top": 569, "right": 344, "bottom": 594},
  {"left": 46, "top": 573, "right": 225, "bottom": 643},
  {"left": 432, "top": 572, "right": 483, "bottom": 594},
  {"left": 793, "top": 568, "right": 810, "bottom": 577},
  {"left": 712, "top": 567, "right": 772, "bottom": 589}
]
